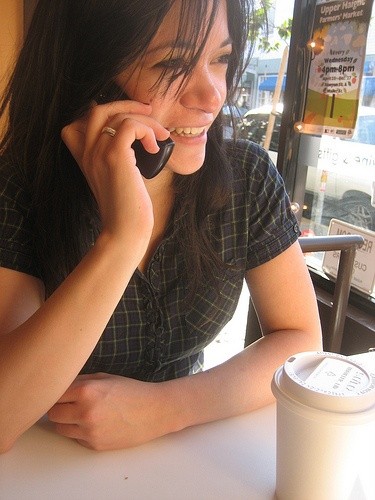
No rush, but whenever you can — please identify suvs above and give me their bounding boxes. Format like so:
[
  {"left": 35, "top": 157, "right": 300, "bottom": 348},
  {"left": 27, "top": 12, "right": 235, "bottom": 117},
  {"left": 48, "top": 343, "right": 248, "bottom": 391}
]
[{"left": 222, "top": 105, "right": 375, "bottom": 232}]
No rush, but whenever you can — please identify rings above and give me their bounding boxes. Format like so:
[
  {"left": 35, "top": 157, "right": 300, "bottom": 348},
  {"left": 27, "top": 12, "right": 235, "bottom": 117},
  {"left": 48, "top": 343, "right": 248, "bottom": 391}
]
[{"left": 101, "top": 126, "right": 116, "bottom": 137}]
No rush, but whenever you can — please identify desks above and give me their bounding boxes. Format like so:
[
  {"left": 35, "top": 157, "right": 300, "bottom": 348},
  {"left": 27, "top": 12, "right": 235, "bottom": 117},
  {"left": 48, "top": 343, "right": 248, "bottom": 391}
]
[{"left": 0, "top": 352, "right": 375, "bottom": 500}]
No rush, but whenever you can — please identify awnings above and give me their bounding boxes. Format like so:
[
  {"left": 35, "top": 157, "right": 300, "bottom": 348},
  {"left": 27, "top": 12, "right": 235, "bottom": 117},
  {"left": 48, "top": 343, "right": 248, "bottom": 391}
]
[{"left": 257, "top": 74, "right": 375, "bottom": 102}]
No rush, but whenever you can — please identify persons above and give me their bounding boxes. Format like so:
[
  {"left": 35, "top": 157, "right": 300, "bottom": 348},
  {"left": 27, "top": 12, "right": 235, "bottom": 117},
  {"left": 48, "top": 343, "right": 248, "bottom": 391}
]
[{"left": 0, "top": 1, "right": 324, "bottom": 459}]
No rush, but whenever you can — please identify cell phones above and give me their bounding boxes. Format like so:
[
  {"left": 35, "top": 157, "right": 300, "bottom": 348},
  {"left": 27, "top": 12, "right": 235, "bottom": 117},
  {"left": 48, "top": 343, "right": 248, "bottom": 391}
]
[{"left": 96, "top": 81, "right": 175, "bottom": 180}]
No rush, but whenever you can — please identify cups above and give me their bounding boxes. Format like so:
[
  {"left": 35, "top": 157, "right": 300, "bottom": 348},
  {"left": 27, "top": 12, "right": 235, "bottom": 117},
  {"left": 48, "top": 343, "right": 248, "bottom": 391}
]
[{"left": 271, "top": 350, "right": 375, "bottom": 500}]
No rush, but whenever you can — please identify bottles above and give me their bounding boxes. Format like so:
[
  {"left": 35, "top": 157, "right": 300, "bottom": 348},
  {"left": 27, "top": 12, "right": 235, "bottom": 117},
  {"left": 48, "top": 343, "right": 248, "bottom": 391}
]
[{"left": 320, "top": 170, "right": 328, "bottom": 192}]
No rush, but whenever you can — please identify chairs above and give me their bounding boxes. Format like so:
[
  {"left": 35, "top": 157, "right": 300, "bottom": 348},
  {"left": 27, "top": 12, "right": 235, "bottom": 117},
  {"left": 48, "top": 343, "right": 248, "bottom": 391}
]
[{"left": 289, "top": 234, "right": 364, "bottom": 356}]
